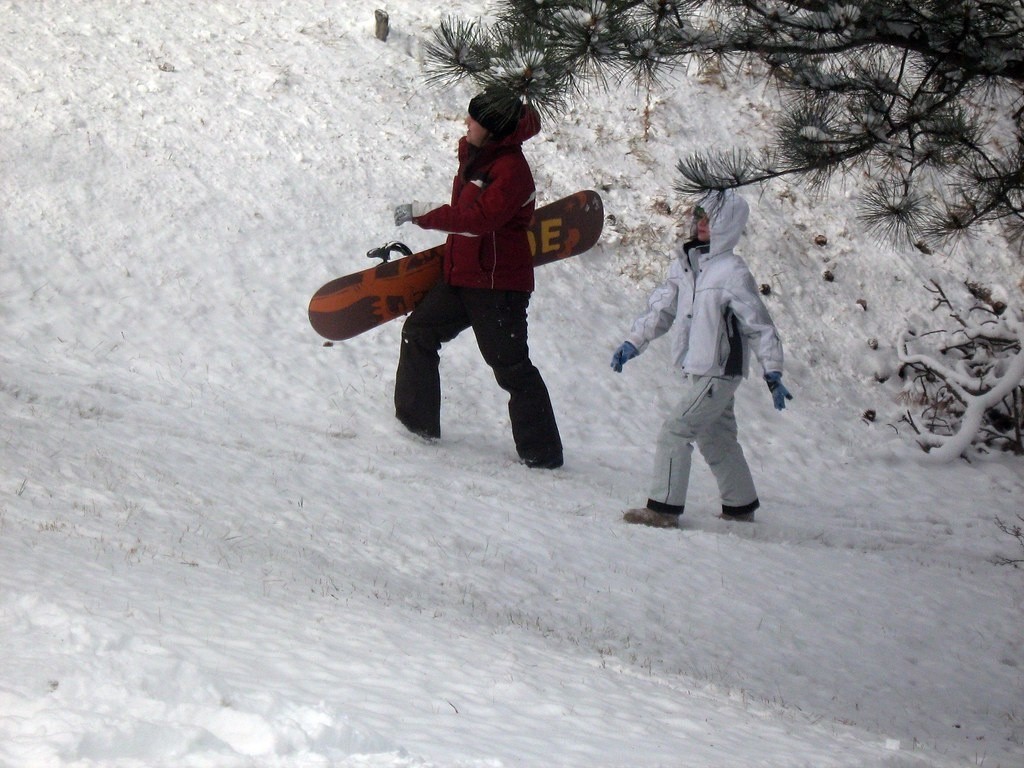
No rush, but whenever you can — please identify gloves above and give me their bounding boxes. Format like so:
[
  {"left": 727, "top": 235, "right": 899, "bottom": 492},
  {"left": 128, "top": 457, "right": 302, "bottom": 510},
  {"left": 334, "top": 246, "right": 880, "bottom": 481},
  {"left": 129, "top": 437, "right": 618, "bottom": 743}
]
[
  {"left": 762, "top": 374, "right": 793, "bottom": 410},
  {"left": 610, "top": 341, "right": 640, "bottom": 373},
  {"left": 394, "top": 203, "right": 412, "bottom": 227}
]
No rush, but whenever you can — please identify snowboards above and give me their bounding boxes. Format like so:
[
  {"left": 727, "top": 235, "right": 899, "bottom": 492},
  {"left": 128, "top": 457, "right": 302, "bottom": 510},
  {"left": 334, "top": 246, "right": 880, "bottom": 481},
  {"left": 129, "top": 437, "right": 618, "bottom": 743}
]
[{"left": 306, "top": 188, "right": 606, "bottom": 340}]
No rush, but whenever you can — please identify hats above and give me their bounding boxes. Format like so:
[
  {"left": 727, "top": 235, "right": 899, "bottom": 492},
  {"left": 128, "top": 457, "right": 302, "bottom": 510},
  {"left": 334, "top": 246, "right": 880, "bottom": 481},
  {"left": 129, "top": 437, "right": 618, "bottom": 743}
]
[{"left": 468, "top": 88, "right": 524, "bottom": 134}]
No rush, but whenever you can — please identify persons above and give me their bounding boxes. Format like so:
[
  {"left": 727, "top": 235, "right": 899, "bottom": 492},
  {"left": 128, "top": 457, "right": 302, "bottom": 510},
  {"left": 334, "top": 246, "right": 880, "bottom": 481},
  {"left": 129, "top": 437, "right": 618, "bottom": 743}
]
[
  {"left": 610, "top": 193, "right": 793, "bottom": 529},
  {"left": 394, "top": 86, "right": 564, "bottom": 469}
]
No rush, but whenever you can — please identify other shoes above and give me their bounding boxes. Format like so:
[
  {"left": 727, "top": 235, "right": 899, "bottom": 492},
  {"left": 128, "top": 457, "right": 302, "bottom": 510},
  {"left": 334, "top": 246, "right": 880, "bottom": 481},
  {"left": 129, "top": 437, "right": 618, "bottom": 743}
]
[
  {"left": 518, "top": 453, "right": 564, "bottom": 471},
  {"left": 623, "top": 506, "right": 681, "bottom": 529},
  {"left": 716, "top": 510, "right": 754, "bottom": 523},
  {"left": 400, "top": 419, "right": 440, "bottom": 441}
]
